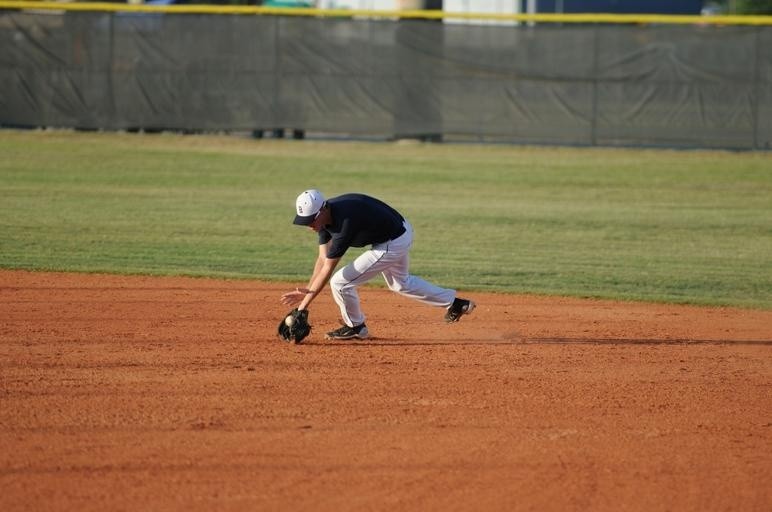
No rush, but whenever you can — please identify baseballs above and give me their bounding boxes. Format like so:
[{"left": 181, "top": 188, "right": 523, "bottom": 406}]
[{"left": 284, "top": 315, "right": 297, "bottom": 327}]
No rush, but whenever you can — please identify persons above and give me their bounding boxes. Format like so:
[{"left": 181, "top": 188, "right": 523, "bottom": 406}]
[
  {"left": 0, "top": 0, "right": 446, "bottom": 147},
  {"left": 279, "top": 188, "right": 475, "bottom": 345}
]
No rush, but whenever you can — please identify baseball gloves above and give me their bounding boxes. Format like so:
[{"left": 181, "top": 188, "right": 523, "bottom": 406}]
[{"left": 277, "top": 309, "right": 311, "bottom": 343}]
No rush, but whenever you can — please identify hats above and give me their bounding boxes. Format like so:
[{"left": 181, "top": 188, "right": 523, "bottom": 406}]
[{"left": 293, "top": 189, "right": 326, "bottom": 226}]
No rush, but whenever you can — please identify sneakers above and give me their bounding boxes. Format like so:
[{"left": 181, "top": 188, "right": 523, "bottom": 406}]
[
  {"left": 324, "top": 322, "right": 370, "bottom": 340},
  {"left": 443, "top": 298, "right": 474, "bottom": 324}
]
